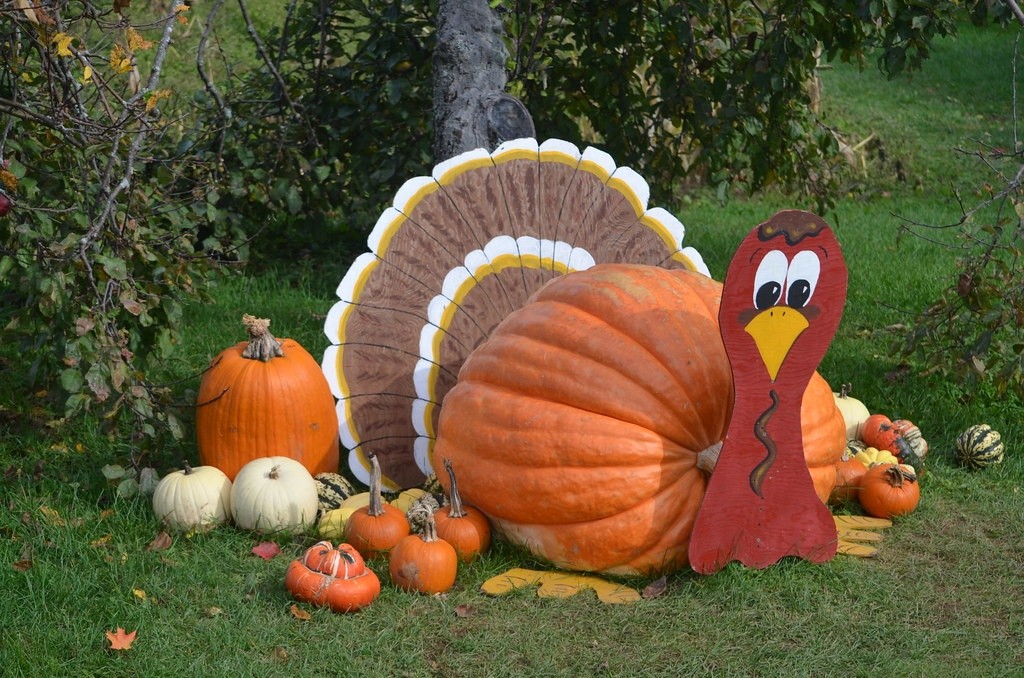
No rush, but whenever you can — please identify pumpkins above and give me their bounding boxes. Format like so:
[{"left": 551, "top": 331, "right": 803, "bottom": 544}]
[
  {"left": 151, "top": 317, "right": 488, "bottom": 611},
  {"left": 432, "top": 264, "right": 848, "bottom": 577},
  {"left": 829, "top": 384, "right": 1003, "bottom": 519}
]
